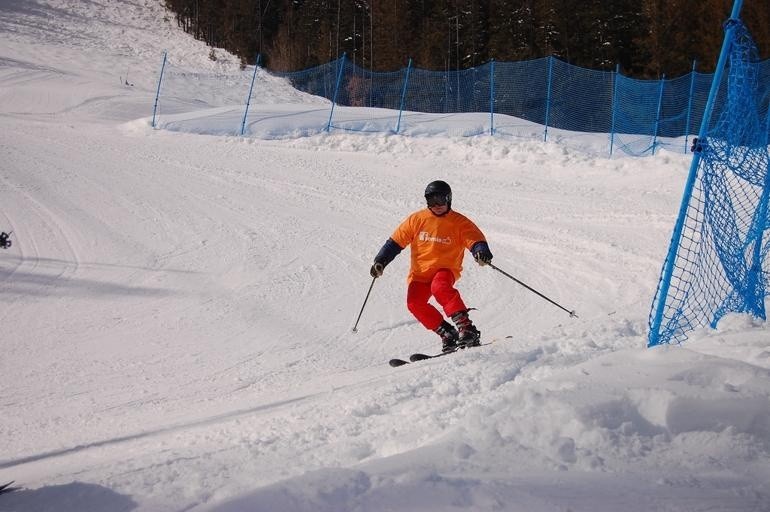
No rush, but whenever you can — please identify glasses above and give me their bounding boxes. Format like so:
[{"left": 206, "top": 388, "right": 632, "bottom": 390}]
[{"left": 427, "top": 192, "right": 452, "bottom": 206}]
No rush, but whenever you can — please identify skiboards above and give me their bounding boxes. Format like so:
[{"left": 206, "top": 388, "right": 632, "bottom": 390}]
[{"left": 389, "top": 337, "right": 512, "bottom": 367}]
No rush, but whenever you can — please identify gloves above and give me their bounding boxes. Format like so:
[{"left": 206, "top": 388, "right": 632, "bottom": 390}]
[
  {"left": 370, "top": 262, "right": 384, "bottom": 278},
  {"left": 472, "top": 251, "right": 491, "bottom": 264}
]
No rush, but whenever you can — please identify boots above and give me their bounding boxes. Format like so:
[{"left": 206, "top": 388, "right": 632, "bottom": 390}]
[
  {"left": 434, "top": 320, "right": 458, "bottom": 346},
  {"left": 452, "top": 311, "right": 477, "bottom": 344}
]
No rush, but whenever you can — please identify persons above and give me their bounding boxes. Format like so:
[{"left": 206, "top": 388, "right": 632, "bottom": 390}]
[{"left": 369, "top": 178, "right": 496, "bottom": 353}]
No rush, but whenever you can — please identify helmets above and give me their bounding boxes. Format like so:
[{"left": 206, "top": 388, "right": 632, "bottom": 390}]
[{"left": 425, "top": 181, "right": 451, "bottom": 196}]
